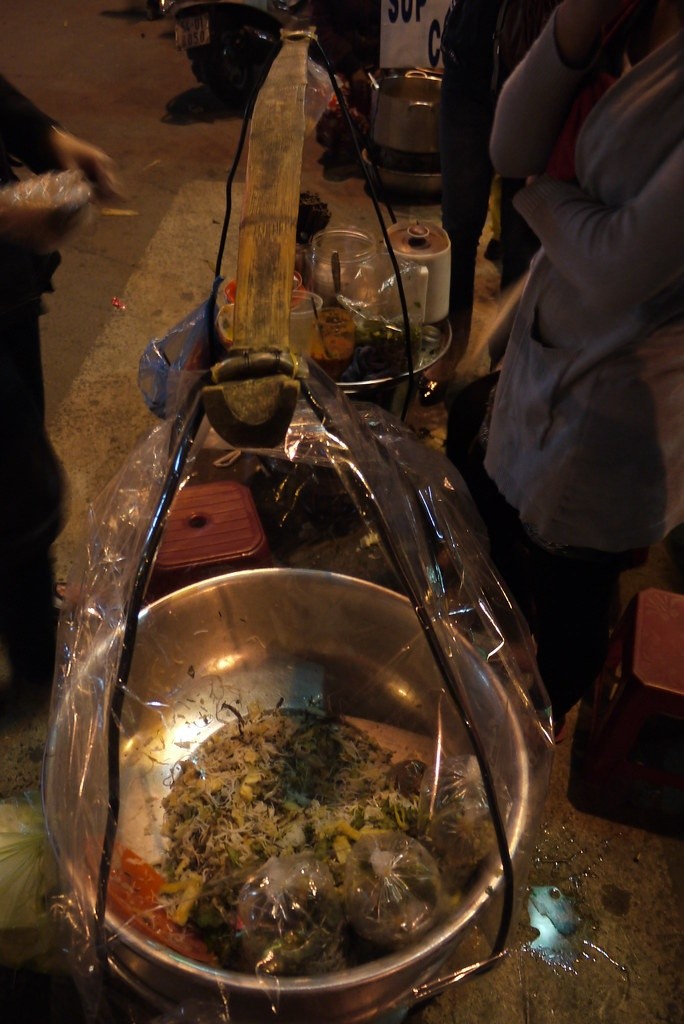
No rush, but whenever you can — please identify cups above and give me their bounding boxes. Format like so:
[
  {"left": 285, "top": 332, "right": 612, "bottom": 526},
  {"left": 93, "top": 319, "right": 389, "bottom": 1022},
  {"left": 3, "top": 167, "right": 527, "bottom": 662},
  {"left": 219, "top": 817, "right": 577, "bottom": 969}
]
[
  {"left": 310, "top": 227, "right": 377, "bottom": 310},
  {"left": 384, "top": 221, "right": 451, "bottom": 324},
  {"left": 289, "top": 290, "right": 323, "bottom": 350}
]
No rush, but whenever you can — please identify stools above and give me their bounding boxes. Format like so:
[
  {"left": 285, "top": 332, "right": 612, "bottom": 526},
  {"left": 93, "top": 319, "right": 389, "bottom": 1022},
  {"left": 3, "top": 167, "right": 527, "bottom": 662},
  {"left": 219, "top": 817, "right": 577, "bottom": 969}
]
[
  {"left": 571, "top": 589, "right": 684, "bottom": 815},
  {"left": 140, "top": 483, "right": 274, "bottom": 607}
]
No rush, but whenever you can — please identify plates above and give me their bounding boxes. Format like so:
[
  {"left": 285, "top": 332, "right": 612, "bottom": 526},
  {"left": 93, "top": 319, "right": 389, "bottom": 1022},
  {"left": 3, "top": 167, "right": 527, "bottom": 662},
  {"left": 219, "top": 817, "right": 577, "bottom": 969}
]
[{"left": 335, "top": 317, "right": 453, "bottom": 395}]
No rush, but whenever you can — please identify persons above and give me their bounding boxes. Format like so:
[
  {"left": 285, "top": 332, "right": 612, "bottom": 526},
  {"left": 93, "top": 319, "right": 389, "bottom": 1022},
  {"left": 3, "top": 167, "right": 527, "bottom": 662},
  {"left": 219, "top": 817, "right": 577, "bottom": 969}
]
[
  {"left": 446, "top": 0, "right": 684, "bottom": 788},
  {"left": 418, "top": 0, "right": 562, "bottom": 408},
  {"left": 0, "top": 72, "right": 131, "bottom": 695}
]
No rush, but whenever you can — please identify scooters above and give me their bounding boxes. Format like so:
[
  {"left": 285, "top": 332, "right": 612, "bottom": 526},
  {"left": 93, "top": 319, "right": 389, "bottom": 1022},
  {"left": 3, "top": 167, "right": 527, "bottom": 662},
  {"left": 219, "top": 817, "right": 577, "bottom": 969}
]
[{"left": 160, "top": 0, "right": 284, "bottom": 111}]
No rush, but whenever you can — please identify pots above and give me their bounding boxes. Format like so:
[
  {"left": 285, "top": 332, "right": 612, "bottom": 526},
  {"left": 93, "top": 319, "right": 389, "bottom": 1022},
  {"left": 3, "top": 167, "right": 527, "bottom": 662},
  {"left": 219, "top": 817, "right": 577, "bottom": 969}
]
[{"left": 368, "top": 71, "right": 442, "bottom": 174}]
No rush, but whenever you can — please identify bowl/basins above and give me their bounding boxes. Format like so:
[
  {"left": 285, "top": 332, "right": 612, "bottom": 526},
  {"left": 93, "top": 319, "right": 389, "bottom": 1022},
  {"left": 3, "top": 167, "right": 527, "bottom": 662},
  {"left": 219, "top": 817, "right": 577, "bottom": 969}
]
[
  {"left": 44, "top": 566, "right": 530, "bottom": 1024},
  {"left": 363, "top": 148, "right": 443, "bottom": 196}
]
